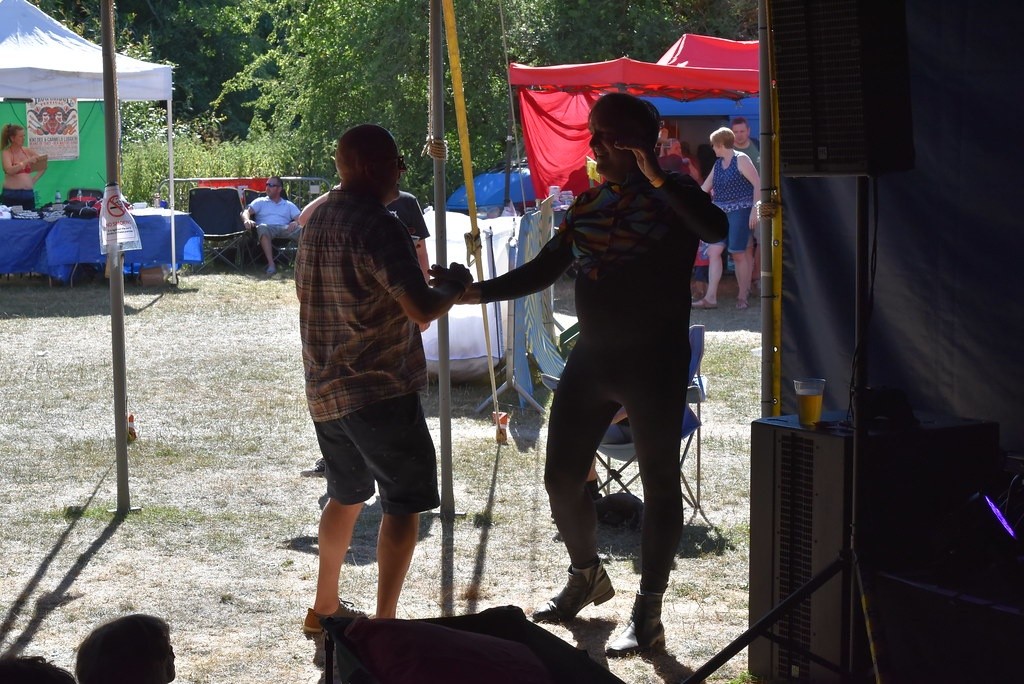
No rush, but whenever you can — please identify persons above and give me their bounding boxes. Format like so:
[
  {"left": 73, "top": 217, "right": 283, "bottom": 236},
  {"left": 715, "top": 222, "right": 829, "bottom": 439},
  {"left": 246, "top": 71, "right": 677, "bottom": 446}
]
[
  {"left": 0, "top": 124, "right": 48, "bottom": 211},
  {"left": 240, "top": 177, "right": 302, "bottom": 275},
  {"left": 585, "top": 405, "right": 627, "bottom": 500},
  {"left": 428, "top": 93, "right": 729, "bottom": 656},
  {"left": 76, "top": 614, "right": 175, "bottom": 684},
  {"left": 0, "top": 654, "right": 77, "bottom": 684},
  {"left": 296, "top": 125, "right": 474, "bottom": 633},
  {"left": 657, "top": 117, "right": 760, "bottom": 310}
]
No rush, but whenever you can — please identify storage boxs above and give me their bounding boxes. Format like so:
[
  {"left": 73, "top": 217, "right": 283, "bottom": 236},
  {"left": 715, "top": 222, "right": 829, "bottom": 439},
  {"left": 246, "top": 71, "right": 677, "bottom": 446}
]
[{"left": 140, "top": 265, "right": 165, "bottom": 290}]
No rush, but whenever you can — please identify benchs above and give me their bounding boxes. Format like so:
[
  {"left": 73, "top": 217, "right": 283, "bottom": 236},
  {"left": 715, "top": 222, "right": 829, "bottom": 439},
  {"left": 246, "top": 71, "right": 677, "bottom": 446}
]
[{"left": 314, "top": 605, "right": 624, "bottom": 684}]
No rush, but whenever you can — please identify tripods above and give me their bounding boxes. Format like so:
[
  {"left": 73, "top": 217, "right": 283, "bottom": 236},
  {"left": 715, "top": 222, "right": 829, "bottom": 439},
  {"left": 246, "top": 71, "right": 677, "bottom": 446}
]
[{"left": 673, "top": 173, "right": 898, "bottom": 684}]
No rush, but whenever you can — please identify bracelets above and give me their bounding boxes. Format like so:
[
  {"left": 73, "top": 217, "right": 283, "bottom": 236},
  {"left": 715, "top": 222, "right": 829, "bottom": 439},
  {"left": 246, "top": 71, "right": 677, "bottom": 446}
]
[
  {"left": 649, "top": 171, "right": 666, "bottom": 188},
  {"left": 446, "top": 278, "right": 467, "bottom": 301}
]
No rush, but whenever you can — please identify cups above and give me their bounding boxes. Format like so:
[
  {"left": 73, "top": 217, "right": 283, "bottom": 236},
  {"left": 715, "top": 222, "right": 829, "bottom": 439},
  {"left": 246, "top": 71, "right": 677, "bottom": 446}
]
[
  {"left": 793, "top": 378, "right": 826, "bottom": 428},
  {"left": 13, "top": 206, "right": 23, "bottom": 214},
  {"left": 154, "top": 193, "right": 160, "bottom": 207}
]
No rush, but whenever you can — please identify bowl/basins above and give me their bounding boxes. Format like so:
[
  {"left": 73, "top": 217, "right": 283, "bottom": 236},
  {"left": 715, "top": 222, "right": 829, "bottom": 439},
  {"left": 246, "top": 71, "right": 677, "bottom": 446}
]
[{"left": 43, "top": 211, "right": 65, "bottom": 218}]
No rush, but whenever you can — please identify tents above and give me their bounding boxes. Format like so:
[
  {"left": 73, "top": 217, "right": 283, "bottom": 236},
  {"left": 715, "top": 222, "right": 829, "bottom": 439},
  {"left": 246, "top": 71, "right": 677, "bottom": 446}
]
[
  {"left": 0, "top": 0, "right": 176, "bottom": 286},
  {"left": 509, "top": 34, "right": 760, "bottom": 208}
]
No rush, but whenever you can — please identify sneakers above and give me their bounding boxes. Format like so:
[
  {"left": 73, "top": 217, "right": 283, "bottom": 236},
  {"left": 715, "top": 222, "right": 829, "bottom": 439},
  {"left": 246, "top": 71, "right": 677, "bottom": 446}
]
[{"left": 303, "top": 598, "right": 368, "bottom": 633}]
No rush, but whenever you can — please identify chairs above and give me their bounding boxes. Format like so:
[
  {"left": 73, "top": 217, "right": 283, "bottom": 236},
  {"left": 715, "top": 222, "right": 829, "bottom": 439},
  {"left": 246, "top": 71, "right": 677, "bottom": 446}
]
[
  {"left": 531, "top": 324, "right": 706, "bottom": 525},
  {"left": 243, "top": 188, "right": 299, "bottom": 273},
  {"left": 188, "top": 186, "right": 250, "bottom": 276},
  {"left": 69, "top": 189, "right": 103, "bottom": 199}
]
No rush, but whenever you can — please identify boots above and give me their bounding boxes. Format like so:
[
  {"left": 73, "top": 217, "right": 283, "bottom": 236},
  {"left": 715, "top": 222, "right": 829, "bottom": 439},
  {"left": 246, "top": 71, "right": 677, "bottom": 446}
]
[
  {"left": 605, "top": 591, "right": 666, "bottom": 657},
  {"left": 533, "top": 556, "right": 614, "bottom": 621}
]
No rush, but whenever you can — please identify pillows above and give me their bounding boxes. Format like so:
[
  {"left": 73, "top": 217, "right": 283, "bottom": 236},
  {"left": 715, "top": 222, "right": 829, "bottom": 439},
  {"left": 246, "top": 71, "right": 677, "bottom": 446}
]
[{"left": 341, "top": 615, "right": 555, "bottom": 684}]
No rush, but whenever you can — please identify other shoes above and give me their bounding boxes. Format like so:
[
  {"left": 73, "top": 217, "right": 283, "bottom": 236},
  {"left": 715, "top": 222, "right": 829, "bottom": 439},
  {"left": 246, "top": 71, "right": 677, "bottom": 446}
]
[{"left": 266, "top": 267, "right": 277, "bottom": 274}]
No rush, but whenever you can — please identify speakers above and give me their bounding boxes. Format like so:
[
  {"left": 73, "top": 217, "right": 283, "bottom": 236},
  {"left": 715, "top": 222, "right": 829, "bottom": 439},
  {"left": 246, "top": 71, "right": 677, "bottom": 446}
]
[
  {"left": 748, "top": 410, "right": 1001, "bottom": 684},
  {"left": 768, "top": 0, "right": 917, "bottom": 182}
]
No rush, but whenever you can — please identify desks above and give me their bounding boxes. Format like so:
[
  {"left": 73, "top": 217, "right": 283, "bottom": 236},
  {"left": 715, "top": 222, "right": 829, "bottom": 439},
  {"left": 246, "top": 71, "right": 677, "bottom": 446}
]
[
  {"left": 0, "top": 209, "right": 64, "bottom": 288},
  {"left": 45, "top": 206, "right": 205, "bottom": 290}
]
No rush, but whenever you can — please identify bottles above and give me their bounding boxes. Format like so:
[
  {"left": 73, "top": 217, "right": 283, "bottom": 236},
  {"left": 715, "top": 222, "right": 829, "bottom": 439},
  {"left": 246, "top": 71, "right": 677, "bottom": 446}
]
[
  {"left": 161, "top": 198, "right": 167, "bottom": 207},
  {"left": 77, "top": 189, "right": 82, "bottom": 202},
  {"left": 55, "top": 190, "right": 61, "bottom": 204}
]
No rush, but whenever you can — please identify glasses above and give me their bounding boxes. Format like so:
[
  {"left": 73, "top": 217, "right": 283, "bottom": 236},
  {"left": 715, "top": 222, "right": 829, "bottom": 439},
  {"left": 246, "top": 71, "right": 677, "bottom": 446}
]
[
  {"left": 372, "top": 154, "right": 405, "bottom": 168},
  {"left": 264, "top": 183, "right": 280, "bottom": 189}
]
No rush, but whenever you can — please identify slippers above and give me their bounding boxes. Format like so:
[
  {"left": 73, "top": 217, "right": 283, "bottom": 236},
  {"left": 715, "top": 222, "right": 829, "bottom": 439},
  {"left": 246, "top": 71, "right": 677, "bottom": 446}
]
[
  {"left": 692, "top": 299, "right": 717, "bottom": 308},
  {"left": 734, "top": 297, "right": 749, "bottom": 309}
]
